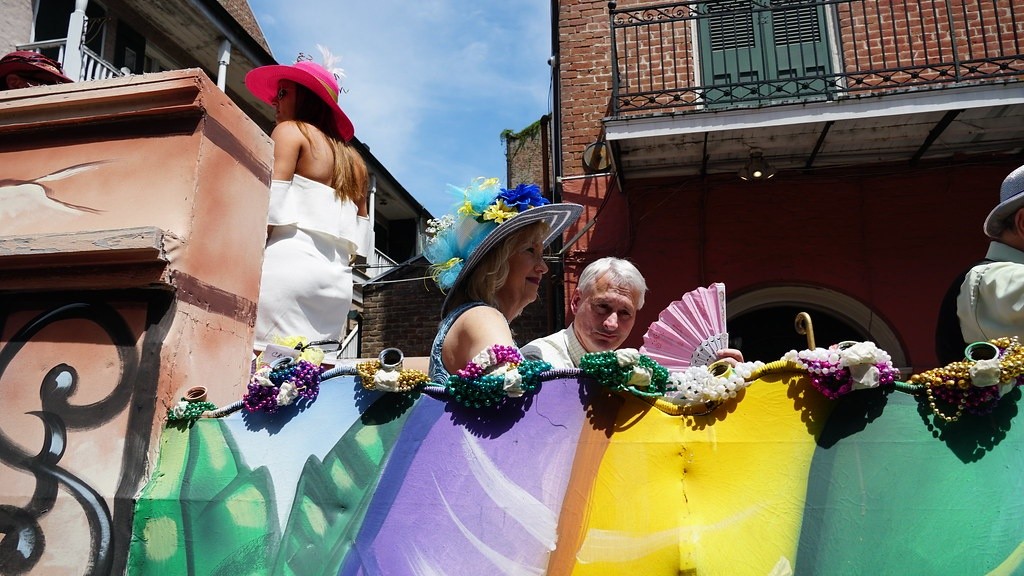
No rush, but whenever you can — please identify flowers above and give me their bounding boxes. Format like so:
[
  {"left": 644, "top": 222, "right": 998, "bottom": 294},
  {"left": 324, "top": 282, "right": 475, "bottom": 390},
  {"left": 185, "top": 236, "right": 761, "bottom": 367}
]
[{"left": 483, "top": 182, "right": 551, "bottom": 223}]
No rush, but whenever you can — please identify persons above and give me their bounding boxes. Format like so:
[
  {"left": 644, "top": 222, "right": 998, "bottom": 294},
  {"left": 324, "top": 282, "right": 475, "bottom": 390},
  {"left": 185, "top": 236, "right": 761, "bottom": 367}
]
[
  {"left": 937, "top": 165, "right": 1024, "bottom": 364},
  {"left": 420, "top": 173, "right": 584, "bottom": 381},
  {"left": 244, "top": 60, "right": 370, "bottom": 360},
  {"left": 518, "top": 256, "right": 744, "bottom": 370}
]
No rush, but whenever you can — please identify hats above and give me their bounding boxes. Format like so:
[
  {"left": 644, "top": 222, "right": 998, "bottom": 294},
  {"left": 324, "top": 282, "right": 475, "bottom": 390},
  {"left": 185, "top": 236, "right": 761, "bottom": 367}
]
[
  {"left": 982, "top": 163, "right": 1024, "bottom": 238},
  {"left": 420, "top": 177, "right": 584, "bottom": 318},
  {"left": 244, "top": 43, "right": 354, "bottom": 142}
]
